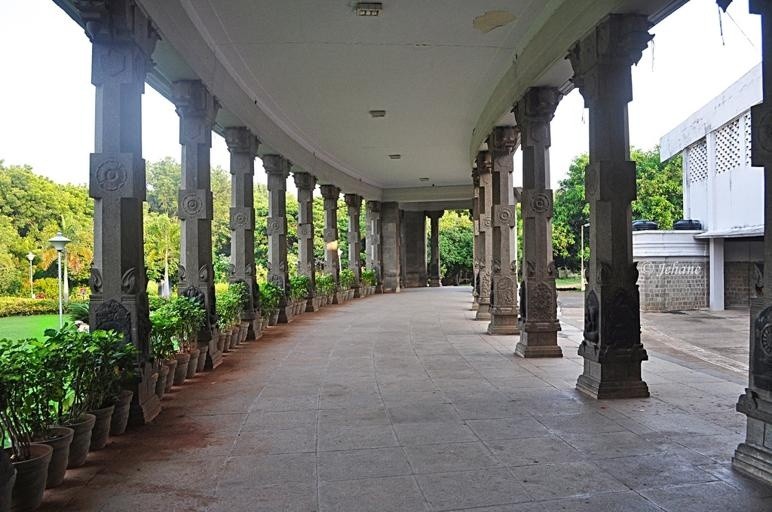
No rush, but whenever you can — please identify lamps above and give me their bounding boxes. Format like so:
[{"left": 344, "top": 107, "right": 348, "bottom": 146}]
[{"left": 354, "top": 4, "right": 383, "bottom": 16}]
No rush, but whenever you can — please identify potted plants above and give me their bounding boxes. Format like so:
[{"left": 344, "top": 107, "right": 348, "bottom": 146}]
[{"left": 0, "top": 267, "right": 377, "bottom": 512}]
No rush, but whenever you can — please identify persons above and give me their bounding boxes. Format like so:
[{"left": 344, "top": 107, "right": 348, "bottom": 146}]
[{"left": 74, "top": 320, "right": 89, "bottom": 334}]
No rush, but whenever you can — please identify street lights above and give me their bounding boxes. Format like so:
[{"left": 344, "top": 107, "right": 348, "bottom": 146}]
[
  {"left": 580, "top": 222, "right": 590, "bottom": 291},
  {"left": 47, "top": 231, "right": 72, "bottom": 330},
  {"left": 25, "top": 252, "right": 36, "bottom": 299}
]
